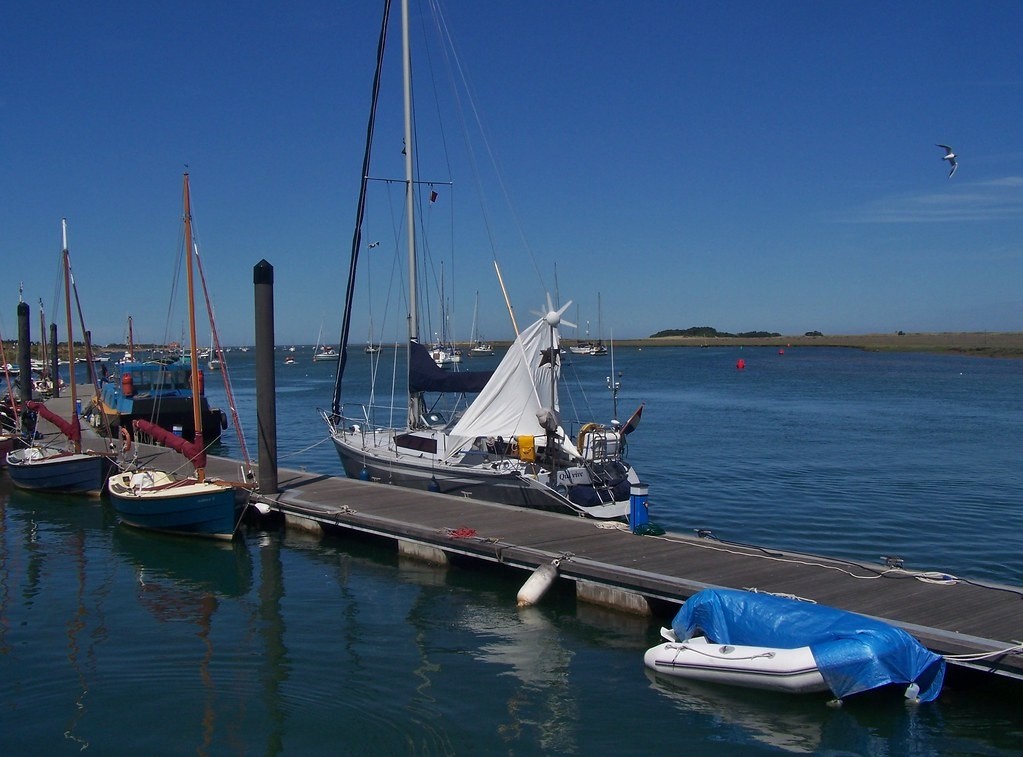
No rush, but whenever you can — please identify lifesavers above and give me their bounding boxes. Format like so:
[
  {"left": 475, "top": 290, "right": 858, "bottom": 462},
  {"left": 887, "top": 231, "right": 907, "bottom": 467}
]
[
  {"left": 576, "top": 422, "right": 605, "bottom": 457},
  {"left": 220, "top": 411, "right": 228, "bottom": 430},
  {"left": 119, "top": 428, "right": 131, "bottom": 452}
]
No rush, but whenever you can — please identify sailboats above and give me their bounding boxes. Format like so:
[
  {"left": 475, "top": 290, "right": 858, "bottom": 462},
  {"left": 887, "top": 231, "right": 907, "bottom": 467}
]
[
  {"left": 106, "top": 165, "right": 257, "bottom": 543},
  {"left": 5, "top": 217, "right": 119, "bottom": 498},
  {"left": 318, "top": 0, "right": 647, "bottom": 521},
  {"left": 206, "top": 305, "right": 228, "bottom": 370},
  {"left": 466, "top": 289, "right": 495, "bottom": 357},
  {"left": 313, "top": 320, "right": 340, "bottom": 362},
  {"left": 570, "top": 292, "right": 609, "bottom": 355},
  {"left": 0, "top": 335, "right": 22, "bottom": 470}
]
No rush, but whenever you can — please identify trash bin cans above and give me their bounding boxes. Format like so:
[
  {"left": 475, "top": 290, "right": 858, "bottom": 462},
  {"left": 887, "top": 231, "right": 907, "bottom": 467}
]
[
  {"left": 172, "top": 425, "right": 182, "bottom": 454},
  {"left": 629, "top": 483, "right": 649, "bottom": 534},
  {"left": 97, "top": 379, "right": 101, "bottom": 388},
  {"left": 77, "top": 399, "right": 82, "bottom": 415}
]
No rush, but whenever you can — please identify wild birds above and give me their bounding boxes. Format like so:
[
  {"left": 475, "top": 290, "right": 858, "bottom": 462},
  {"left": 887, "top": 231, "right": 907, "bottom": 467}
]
[{"left": 934, "top": 143, "right": 959, "bottom": 180}]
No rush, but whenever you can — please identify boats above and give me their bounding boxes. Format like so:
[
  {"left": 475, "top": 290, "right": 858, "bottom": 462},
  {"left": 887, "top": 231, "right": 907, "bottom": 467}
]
[
  {"left": 283, "top": 345, "right": 295, "bottom": 352},
  {"left": 641, "top": 587, "right": 914, "bottom": 695},
  {"left": 426, "top": 331, "right": 463, "bottom": 370},
  {"left": 86, "top": 315, "right": 228, "bottom": 442},
  {"left": 312, "top": 345, "right": 333, "bottom": 351},
  {"left": 115, "top": 352, "right": 137, "bottom": 366},
  {"left": 282, "top": 356, "right": 296, "bottom": 365},
  {"left": 168, "top": 346, "right": 251, "bottom": 357},
  {"left": 365, "top": 344, "right": 376, "bottom": 353},
  {"left": 0, "top": 354, "right": 111, "bottom": 374}
]
